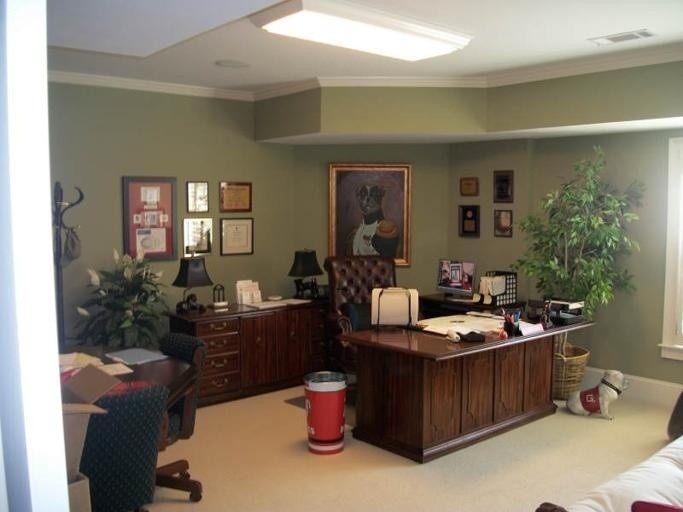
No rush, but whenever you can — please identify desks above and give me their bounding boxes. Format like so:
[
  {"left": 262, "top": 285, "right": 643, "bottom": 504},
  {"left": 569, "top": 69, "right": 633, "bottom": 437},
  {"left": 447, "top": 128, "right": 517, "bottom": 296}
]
[
  {"left": 419, "top": 292, "right": 528, "bottom": 318},
  {"left": 170, "top": 297, "right": 332, "bottom": 409},
  {"left": 336, "top": 300, "right": 596, "bottom": 464},
  {"left": 60, "top": 344, "right": 200, "bottom": 410}
]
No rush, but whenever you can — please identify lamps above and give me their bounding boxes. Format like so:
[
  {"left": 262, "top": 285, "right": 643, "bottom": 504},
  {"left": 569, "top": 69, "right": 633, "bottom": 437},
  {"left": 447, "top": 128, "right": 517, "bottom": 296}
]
[
  {"left": 246, "top": 0, "right": 473, "bottom": 64},
  {"left": 287, "top": 250, "right": 324, "bottom": 299},
  {"left": 171, "top": 256, "right": 213, "bottom": 316}
]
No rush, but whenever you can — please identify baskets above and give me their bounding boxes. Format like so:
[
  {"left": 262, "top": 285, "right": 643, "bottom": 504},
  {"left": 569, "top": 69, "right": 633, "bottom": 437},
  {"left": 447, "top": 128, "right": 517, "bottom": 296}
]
[{"left": 552, "top": 344, "right": 591, "bottom": 400}]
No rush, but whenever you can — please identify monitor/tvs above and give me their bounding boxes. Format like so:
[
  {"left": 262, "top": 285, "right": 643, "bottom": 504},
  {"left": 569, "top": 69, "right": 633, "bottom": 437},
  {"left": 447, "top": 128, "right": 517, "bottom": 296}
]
[{"left": 435, "top": 257, "right": 482, "bottom": 302}]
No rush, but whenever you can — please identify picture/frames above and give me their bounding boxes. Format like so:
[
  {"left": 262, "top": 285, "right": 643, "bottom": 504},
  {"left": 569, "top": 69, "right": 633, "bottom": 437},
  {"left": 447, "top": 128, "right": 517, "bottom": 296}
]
[
  {"left": 219, "top": 218, "right": 254, "bottom": 255},
  {"left": 122, "top": 175, "right": 179, "bottom": 261},
  {"left": 327, "top": 161, "right": 413, "bottom": 270},
  {"left": 459, "top": 177, "right": 480, "bottom": 196},
  {"left": 186, "top": 180, "right": 210, "bottom": 212},
  {"left": 219, "top": 181, "right": 253, "bottom": 211},
  {"left": 493, "top": 170, "right": 515, "bottom": 203},
  {"left": 458, "top": 205, "right": 480, "bottom": 239},
  {"left": 493, "top": 209, "right": 512, "bottom": 238}
]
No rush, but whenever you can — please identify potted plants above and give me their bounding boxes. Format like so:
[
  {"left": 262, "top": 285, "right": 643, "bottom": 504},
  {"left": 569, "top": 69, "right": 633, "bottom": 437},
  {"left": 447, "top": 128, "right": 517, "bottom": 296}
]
[{"left": 511, "top": 146, "right": 647, "bottom": 401}]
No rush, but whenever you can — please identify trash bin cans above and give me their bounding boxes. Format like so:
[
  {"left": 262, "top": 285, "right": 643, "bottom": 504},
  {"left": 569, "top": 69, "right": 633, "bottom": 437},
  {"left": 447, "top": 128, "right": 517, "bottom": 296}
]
[{"left": 302, "top": 371, "right": 348, "bottom": 456}]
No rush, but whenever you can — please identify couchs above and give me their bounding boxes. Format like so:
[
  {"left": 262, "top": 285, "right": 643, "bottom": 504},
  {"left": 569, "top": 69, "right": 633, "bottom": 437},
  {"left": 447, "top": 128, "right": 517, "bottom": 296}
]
[{"left": 537, "top": 393, "right": 683, "bottom": 511}]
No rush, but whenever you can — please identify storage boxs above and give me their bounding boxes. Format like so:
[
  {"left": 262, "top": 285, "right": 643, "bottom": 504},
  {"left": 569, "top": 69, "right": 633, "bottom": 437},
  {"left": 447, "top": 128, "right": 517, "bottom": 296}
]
[
  {"left": 69, "top": 473, "right": 92, "bottom": 511},
  {"left": 62, "top": 363, "right": 121, "bottom": 484}
]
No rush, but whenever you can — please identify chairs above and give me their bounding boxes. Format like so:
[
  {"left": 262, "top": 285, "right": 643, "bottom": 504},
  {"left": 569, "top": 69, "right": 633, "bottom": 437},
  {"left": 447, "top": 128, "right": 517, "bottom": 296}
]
[
  {"left": 324, "top": 255, "right": 396, "bottom": 406},
  {"left": 80, "top": 386, "right": 170, "bottom": 512},
  {"left": 164, "top": 333, "right": 204, "bottom": 445}
]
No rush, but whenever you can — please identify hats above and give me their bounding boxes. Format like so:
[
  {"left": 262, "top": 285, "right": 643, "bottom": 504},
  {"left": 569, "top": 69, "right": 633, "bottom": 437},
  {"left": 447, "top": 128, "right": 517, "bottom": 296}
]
[{"left": 59, "top": 228, "right": 82, "bottom": 267}]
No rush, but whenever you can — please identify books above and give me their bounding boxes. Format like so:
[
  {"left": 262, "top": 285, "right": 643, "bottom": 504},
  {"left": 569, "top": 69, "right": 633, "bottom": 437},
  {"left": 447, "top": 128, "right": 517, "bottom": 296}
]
[{"left": 246, "top": 301, "right": 287, "bottom": 310}]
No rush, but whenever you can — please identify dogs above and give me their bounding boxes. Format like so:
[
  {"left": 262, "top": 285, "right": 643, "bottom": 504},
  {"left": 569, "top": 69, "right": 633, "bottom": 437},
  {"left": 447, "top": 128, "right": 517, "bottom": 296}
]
[{"left": 567, "top": 369, "right": 629, "bottom": 420}]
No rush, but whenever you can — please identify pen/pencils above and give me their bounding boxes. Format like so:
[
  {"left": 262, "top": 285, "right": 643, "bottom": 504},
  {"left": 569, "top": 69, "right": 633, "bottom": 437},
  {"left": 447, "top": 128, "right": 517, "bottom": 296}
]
[{"left": 499, "top": 306, "right": 521, "bottom": 323}]
